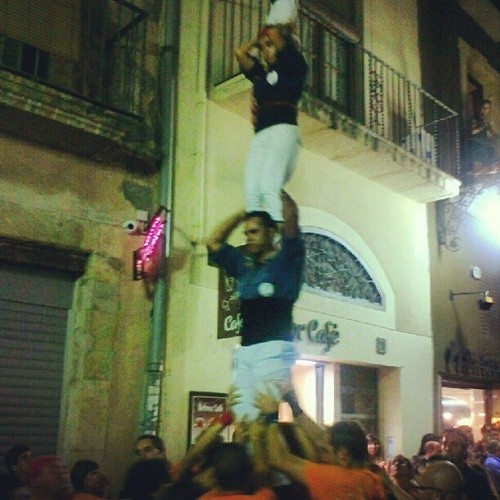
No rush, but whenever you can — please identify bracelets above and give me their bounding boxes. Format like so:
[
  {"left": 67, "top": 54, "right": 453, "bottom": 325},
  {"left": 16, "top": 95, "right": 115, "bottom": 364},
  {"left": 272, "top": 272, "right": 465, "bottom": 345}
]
[
  {"left": 266, "top": 411, "right": 280, "bottom": 424},
  {"left": 213, "top": 410, "right": 235, "bottom": 427}
]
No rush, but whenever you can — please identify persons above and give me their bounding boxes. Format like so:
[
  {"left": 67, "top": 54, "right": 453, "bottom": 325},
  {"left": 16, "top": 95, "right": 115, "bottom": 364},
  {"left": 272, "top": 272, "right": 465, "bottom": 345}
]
[
  {"left": 466, "top": 100, "right": 500, "bottom": 165},
  {"left": 205, "top": 188, "right": 307, "bottom": 486},
  {"left": 0, "top": 373, "right": 500, "bottom": 500},
  {"left": 233, "top": 0, "right": 308, "bottom": 261}
]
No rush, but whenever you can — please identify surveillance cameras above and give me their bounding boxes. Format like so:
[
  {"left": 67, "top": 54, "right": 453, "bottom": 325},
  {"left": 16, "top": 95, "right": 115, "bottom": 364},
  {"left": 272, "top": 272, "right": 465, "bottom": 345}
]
[{"left": 122, "top": 220, "right": 139, "bottom": 234}]
